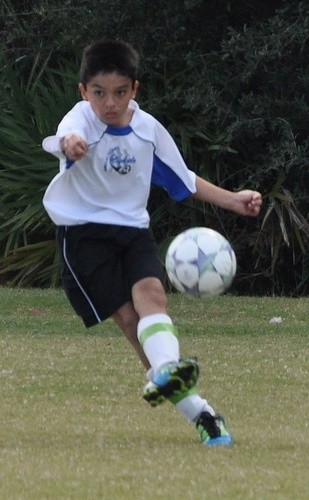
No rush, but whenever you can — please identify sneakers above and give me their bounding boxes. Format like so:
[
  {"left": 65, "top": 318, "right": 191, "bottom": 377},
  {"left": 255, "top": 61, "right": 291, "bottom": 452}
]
[
  {"left": 142, "top": 360, "right": 199, "bottom": 407},
  {"left": 195, "top": 412, "right": 234, "bottom": 447}
]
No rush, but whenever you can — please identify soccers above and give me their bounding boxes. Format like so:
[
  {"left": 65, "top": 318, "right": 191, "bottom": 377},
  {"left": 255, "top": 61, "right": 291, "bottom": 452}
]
[{"left": 165, "top": 228, "right": 236, "bottom": 298}]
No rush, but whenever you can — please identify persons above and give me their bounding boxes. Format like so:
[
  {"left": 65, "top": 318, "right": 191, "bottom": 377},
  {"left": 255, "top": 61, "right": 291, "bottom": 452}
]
[{"left": 40, "top": 40, "right": 262, "bottom": 444}]
